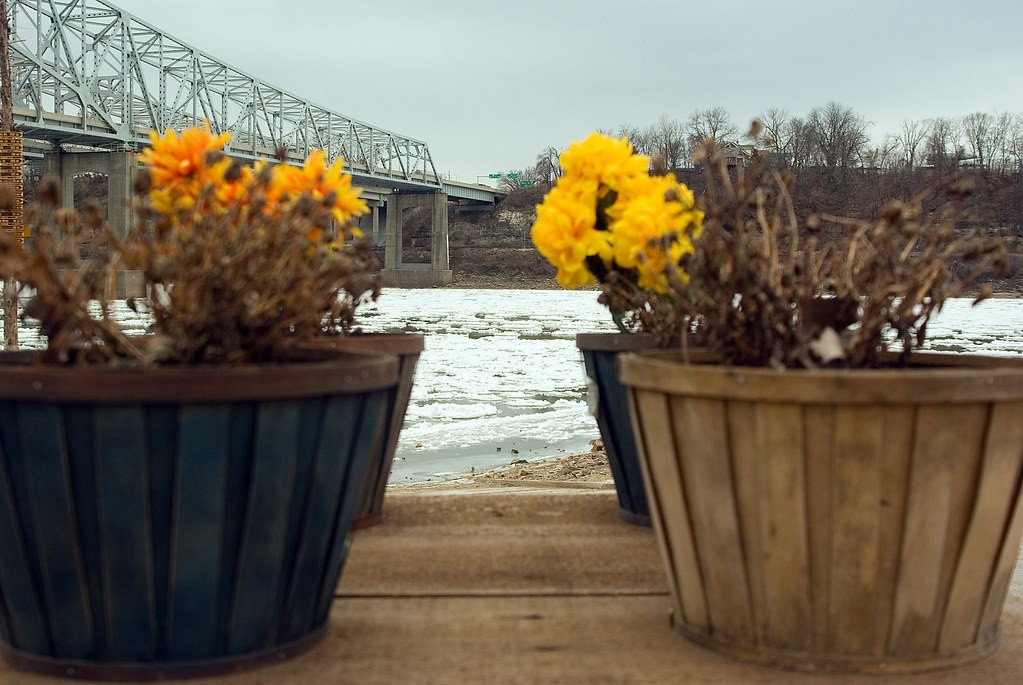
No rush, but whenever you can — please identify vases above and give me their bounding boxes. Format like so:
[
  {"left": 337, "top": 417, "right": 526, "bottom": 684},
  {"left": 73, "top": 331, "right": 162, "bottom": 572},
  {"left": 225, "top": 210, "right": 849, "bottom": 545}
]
[
  {"left": 574, "top": 333, "right": 1023, "bottom": 679},
  {"left": 0, "top": 331, "right": 426, "bottom": 685}
]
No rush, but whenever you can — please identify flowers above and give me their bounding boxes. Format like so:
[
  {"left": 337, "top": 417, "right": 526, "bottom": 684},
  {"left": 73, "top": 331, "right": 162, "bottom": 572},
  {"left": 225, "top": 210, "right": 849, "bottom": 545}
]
[
  {"left": 525, "top": 129, "right": 706, "bottom": 334},
  {"left": 142, "top": 122, "right": 371, "bottom": 259}
]
